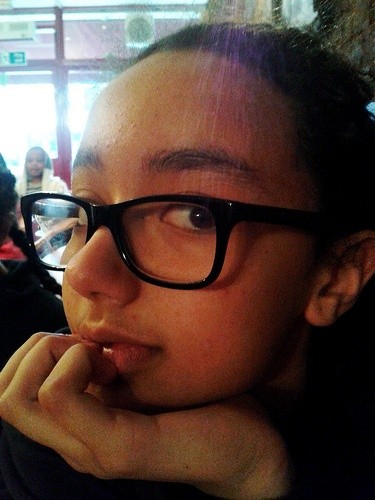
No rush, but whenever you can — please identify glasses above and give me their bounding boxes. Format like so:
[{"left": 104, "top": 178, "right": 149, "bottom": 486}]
[{"left": 19, "top": 193, "right": 332, "bottom": 292}]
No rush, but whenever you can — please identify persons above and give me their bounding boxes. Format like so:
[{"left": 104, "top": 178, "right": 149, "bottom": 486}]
[
  {"left": 0, "top": 170, "right": 80, "bottom": 498},
  {"left": 0, "top": 23, "right": 375, "bottom": 500},
  {"left": 13, "top": 147, "right": 73, "bottom": 200}
]
